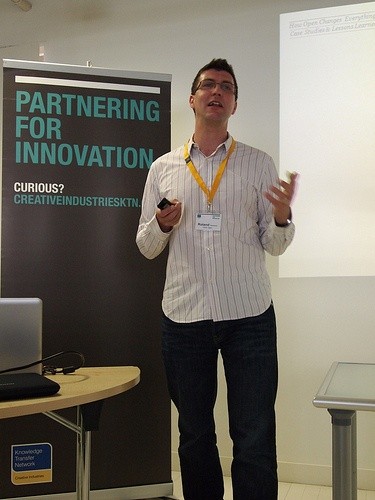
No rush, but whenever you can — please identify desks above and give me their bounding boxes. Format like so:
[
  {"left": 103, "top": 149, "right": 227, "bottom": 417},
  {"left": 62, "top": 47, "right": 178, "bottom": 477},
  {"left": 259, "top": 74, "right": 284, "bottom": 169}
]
[
  {"left": 312, "top": 362, "right": 375, "bottom": 500},
  {"left": 0, "top": 366, "right": 141, "bottom": 500}
]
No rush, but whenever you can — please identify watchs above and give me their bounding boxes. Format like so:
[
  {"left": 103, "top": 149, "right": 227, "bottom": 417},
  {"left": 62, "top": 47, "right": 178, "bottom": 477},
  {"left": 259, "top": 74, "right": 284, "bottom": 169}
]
[{"left": 273, "top": 204, "right": 292, "bottom": 227}]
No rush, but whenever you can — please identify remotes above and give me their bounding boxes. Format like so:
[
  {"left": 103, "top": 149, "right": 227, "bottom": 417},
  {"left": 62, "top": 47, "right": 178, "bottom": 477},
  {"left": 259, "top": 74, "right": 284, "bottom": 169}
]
[{"left": 157, "top": 197, "right": 173, "bottom": 210}]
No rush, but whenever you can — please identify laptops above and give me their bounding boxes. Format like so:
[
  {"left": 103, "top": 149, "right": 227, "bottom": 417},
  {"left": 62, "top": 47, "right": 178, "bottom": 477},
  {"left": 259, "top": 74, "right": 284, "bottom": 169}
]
[{"left": 0, "top": 297, "right": 42, "bottom": 375}]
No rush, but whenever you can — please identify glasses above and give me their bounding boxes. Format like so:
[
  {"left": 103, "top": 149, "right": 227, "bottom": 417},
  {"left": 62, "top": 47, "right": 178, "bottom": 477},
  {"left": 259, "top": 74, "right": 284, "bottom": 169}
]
[{"left": 191, "top": 79, "right": 237, "bottom": 97}]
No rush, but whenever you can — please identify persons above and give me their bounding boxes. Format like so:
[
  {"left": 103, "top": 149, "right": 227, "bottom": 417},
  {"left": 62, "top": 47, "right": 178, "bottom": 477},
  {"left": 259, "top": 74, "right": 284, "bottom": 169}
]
[{"left": 135, "top": 56, "right": 297, "bottom": 500}]
